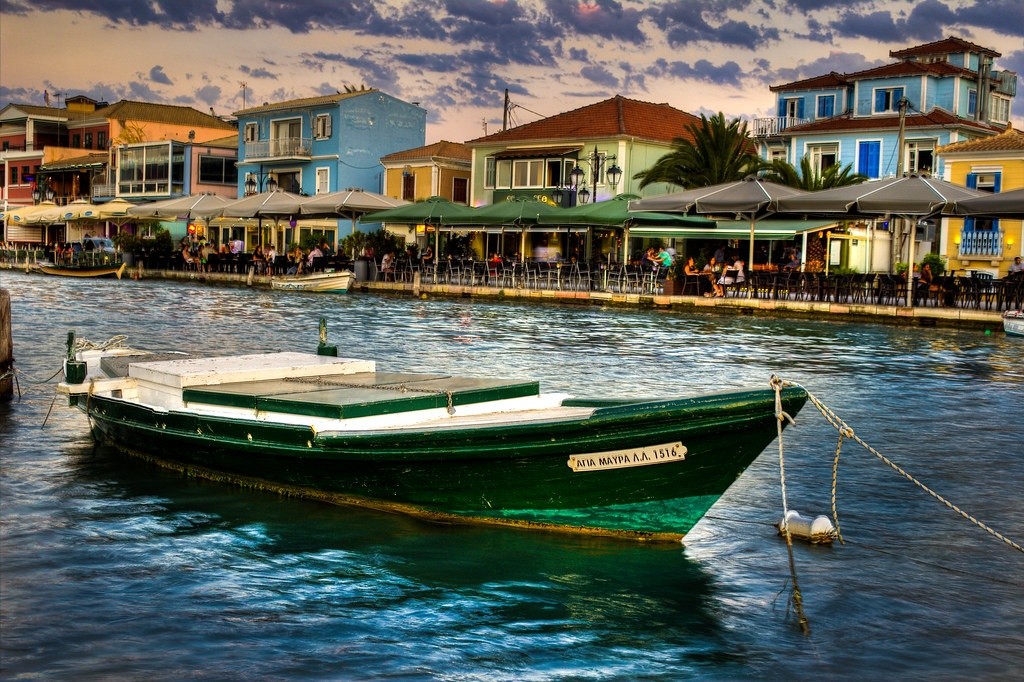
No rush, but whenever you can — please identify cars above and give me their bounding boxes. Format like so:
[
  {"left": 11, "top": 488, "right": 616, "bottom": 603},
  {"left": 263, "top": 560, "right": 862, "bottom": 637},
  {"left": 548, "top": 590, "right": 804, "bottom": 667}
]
[{"left": 83, "top": 237, "right": 118, "bottom": 255}]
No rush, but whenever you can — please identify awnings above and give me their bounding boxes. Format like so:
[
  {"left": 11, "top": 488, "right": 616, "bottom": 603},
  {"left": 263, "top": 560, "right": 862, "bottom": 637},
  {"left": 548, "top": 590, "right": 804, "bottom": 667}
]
[{"left": 628, "top": 219, "right": 836, "bottom": 238}]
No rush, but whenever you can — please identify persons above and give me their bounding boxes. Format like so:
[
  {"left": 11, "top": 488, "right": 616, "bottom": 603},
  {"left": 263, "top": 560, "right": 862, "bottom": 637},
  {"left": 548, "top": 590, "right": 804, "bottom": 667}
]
[
  {"left": 641, "top": 245, "right": 676, "bottom": 279},
  {"left": 1008, "top": 257, "right": 1024, "bottom": 275},
  {"left": 921, "top": 263, "right": 945, "bottom": 306},
  {"left": 683, "top": 243, "right": 746, "bottom": 298},
  {"left": 44, "top": 242, "right": 82, "bottom": 262},
  {"left": 253, "top": 238, "right": 331, "bottom": 276},
  {"left": 381, "top": 244, "right": 433, "bottom": 281},
  {"left": 83, "top": 234, "right": 94, "bottom": 252},
  {"left": 99, "top": 242, "right": 106, "bottom": 251},
  {"left": 913, "top": 263, "right": 927, "bottom": 306},
  {"left": 178, "top": 236, "right": 217, "bottom": 272},
  {"left": 796, "top": 247, "right": 801, "bottom": 260}
]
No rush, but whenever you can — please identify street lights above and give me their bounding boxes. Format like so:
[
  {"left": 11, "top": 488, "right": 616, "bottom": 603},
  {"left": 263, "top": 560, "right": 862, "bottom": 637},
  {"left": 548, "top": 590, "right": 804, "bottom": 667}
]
[
  {"left": 552, "top": 172, "right": 591, "bottom": 261},
  {"left": 31, "top": 174, "right": 55, "bottom": 249},
  {"left": 245, "top": 162, "right": 277, "bottom": 246},
  {"left": 570, "top": 144, "right": 624, "bottom": 290}
]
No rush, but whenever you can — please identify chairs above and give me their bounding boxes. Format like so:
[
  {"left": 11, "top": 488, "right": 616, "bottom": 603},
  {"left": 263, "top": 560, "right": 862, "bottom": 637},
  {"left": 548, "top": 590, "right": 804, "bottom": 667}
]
[{"left": 131, "top": 247, "right": 1024, "bottom": 313}]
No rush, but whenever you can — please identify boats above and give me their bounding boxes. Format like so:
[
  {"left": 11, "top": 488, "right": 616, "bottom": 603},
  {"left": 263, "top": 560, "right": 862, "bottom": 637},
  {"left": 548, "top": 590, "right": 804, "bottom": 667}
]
[
  {"left": 35, "top": 258, "right": 127, "bottom": 280},
  {"left": 269, "top": 270, "right": 357, "bottom": 295},
  {"left": 1002, "top": 310, "right": 1024, "bottom": 337},
  {"left": 62, "top": 318, "right": 808, "bottom": 553}
]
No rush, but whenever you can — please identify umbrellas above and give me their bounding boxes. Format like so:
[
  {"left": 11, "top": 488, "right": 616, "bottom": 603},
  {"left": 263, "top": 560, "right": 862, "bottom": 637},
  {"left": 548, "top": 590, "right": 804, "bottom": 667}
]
[
  {"left": 358, "top": 197, "right": 477, "bottom": 268},
  {"left": 537, "top": 194, "right": 716, "bottom": 268},
  {"left": 259, "top": 186, "right": 412, "bottom": 262},
  {"left": 217, "top": 190, "right": 308, "bottom": 253},
  {"left": 439, "top": 198, "right": 563, "bottom": 277},
  {"left": 0, "top": 197, "right": 137, "bottom": 250},
  {"left": 776, "top": 174, "right": 991, "bottom": 308},
  {"left": 953, "top": 189, "right": 1024, "bottom": 218},
  {"left": 126, "top": 192, "right": 236, "bottom": 242},
  {"left": 628, "top": 177, "right": 809, "bottom": 299}
]
